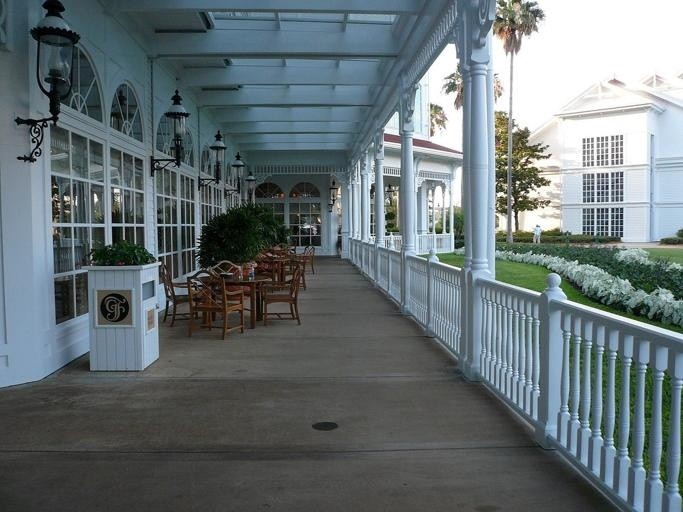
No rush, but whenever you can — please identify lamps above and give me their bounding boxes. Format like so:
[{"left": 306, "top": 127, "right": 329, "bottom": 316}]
[
  {"left": 197, "top": 130, "right": 227, "bottom": 191},
  {"left": 224, "top": 151, "right": 245, "bottom": 199},
  {"left": 328, "top": 179, "right": 338, "bottom": 212},
  {"left": 150, "top": 89, "right": 193, "bottom": 178},
  {"left": 242, "top": 171, "right": 258, "bottom": 202},
  {"left": 385, "top": 184, "right": 394, "bottom": 205},
  {"left": 13, "top": 0, "right": 82, "bottom": 163}
]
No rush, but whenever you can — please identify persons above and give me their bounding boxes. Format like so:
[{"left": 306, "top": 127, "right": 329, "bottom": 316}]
[{"left": 533, "top": 224, "right": 541, "bottom": 244}]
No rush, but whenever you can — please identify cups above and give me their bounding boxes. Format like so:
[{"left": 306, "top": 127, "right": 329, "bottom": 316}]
[
  {"left": 248, "top": 268, "right": 254, "bottom": 279},
  {"left": 238, "top": 271, "right": 243, "bottom": 278}
]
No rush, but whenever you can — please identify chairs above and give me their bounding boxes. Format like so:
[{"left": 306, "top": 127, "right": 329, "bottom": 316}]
[
  {"left": 52, "top": 260, "right": 88, "bottom": 326},
  {"left": 160, "top": 241, "right": 318, "bottom": 341}
]
